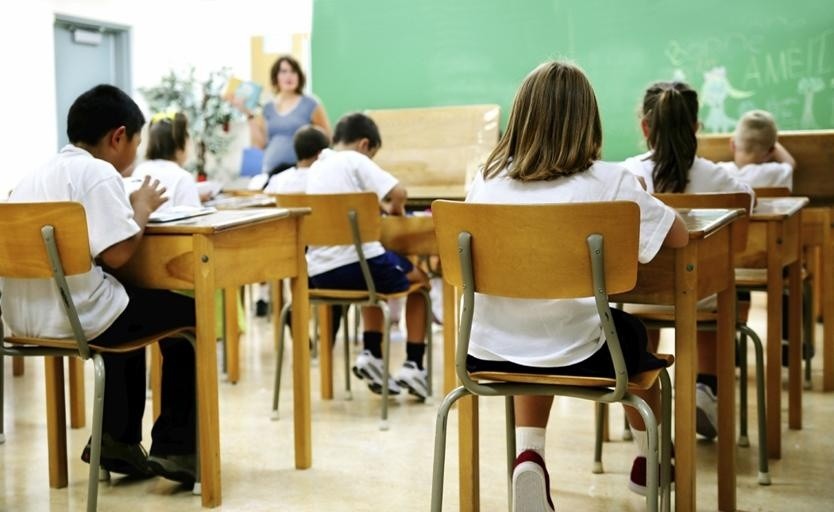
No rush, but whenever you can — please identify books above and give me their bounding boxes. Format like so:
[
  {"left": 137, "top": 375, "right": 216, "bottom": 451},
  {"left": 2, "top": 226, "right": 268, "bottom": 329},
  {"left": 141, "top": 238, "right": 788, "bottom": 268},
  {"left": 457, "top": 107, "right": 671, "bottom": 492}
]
[
  {"left": 146, "top": 206, "right": 217, "bottom": 222},
  {"left": 204, "top": 192, "right": 276, "bottom": 211},
  {"left": 221, "top": 79, "right": 262, "bottom": 114}
]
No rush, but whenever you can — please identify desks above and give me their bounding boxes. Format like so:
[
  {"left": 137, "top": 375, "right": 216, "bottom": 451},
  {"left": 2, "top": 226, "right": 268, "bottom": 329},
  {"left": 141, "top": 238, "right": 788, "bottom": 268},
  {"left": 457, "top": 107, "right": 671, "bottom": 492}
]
[
  {"left": 736, "top": 196, "right": 810, "bottom": 459},
  {"left": 459, "top": 207, "right": 747, "bottom": 510},
  {"left": 383, "top": 187, "right": 469, "bottom": 407},
  {"left": 202, "top": 193, "right": 282, "bottom": 383},
  {"left": 804, "top": 197, "right": 834, "bottom": 391},
  {"left": 121, "top": 207, "right": 312, "bottom": 509}
]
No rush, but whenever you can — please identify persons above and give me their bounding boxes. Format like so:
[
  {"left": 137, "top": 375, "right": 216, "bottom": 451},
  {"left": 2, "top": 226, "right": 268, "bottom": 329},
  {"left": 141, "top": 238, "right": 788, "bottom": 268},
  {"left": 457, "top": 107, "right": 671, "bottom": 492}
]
[
  {"left": 456, "top": 61, "right": 690, "bottom": 511},
  {"left": 303, "top": 114, "right": 435, "bottom": 403},
  {"left": 0, "top": 84, "right": 198, "bottom": 485},
  {"left": 263, "top": 126, "right": 344, "bottom": 358},
  {"left": 126, "top": 110, "right": 214, "bottom": 214},
  {"left": 624, "top": 81, "right": 758, "bottom": 439},
  {"left": 715, "top": 110, "right": 814, "bottom": 389},
  {"left": 232, "top": 55, "right": 331, "bottom": 327}
]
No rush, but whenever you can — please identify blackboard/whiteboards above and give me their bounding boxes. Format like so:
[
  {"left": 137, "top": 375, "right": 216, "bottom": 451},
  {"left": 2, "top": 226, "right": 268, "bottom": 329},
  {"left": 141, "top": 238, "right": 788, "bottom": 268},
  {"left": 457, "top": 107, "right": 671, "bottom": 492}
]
[{"left": 309, "top": 0, "right": 834, "bottom": 166}]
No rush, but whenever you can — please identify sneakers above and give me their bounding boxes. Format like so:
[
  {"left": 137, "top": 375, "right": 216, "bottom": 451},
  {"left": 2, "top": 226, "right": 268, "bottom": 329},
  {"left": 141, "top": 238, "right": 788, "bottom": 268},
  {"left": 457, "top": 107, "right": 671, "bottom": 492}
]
[
  {"left": 695, "top": 382, "right": 718, "bottom": 440},
  {"left": 628, "top": 456, "right": 674, "bottom": 497},
  {"left": 351, "top": 349, "right": 400, "bottom": 396},
  {"left": 145, "top": 452, "right": 197, "bottom": 483},
  {"left": 512, "top": 449, "right": 554, "bottom": 512},
  {"left": 82, "top": 433, "right": 158, "bottom": 477},
  {"left": 396, "top": 361, "right": 429, "bottom": 400}
]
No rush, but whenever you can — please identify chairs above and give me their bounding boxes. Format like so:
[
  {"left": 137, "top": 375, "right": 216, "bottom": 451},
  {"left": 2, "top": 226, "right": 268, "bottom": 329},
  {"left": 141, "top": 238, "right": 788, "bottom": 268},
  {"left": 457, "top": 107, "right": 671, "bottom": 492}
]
[
  {"left": 735, "top": 187, "right": 808, "bottom": 291},
  {"left": 270, "top": 193, "right": 431, "bottom": 432},
  {"left": 432, "top": 200, "right": 674, "bottom": 511},
  {"left": 592, "top": 192, "right": 769, "bottom": 484},
  {"left": 0, "top": 203, "right": 196, "bottom": 512}
]
